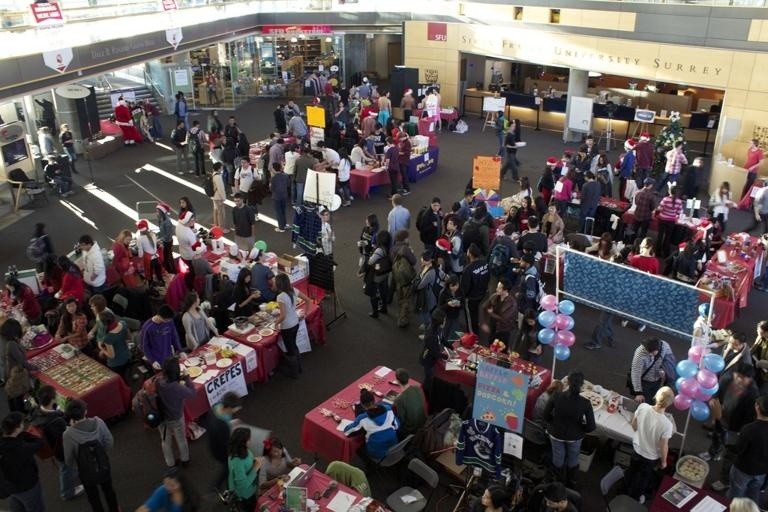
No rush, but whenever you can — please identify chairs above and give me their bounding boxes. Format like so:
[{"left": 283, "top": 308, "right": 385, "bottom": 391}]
[
  {"left": 7, "top": 168, "right": 48, "bottom": 208},
  {"left": 385, "top": 457, "right": 440, "bottom": 511}
]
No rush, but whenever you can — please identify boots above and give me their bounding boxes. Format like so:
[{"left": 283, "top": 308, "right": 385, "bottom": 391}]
[{"left": 369, "top": 295, "right": 387, "bottom": 318}]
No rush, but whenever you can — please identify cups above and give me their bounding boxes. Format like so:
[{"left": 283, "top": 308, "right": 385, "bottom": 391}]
[
  {"left": 507, "top": 415, "right": 519, "bottom": 431},
  {"left": 328, "top": 479, "right": 338, "bottom": 491},
  {"left": 728, "top": 159, "right": 733, "bottom": 165},
  {"left": 354, "top": 162, "right": 362, "bottom": 171}
]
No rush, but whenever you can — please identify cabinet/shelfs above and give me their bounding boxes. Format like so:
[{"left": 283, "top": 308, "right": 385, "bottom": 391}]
[{"left": 276, "top": 39, "right": 322, "bottom": 63}]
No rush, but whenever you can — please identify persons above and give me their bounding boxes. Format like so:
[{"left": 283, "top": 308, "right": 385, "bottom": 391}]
[
  {"left": 583, "top": 255, "right": 628, "bottom": 351},
  {"left": 705, "top": 320, "right": 766, "bottom": 510},
  {"left": 535, "top": 371, "right": 595, "bottom": 489},
  {"left": 140, "top": 102, "right": 163, "bottom": 141},
  {"left": 157, "top": 76, "right": 441, "bottom": 234},
  {"left": 532, "top": 482, "right": 576, "bottom": 510},
  {"left": 344, "top": 387, "right": 401, "bottom": 467},
  {"left": 492, "top": 110, "right": 523, "bottom": 182},
  {"left": 628, "top": 386, "right": 675, "bottom": 505},
  {"left": 543, "top": 133, "right": 767, "bottom": 287},
  {"left": 360, "top": 176, "right": 566, "bottom": 380},
  {"left": 630, "top": 335, "right": 672, "bottom": 404},
  {"left": 394, "top": 369, "right": 427, "bottom": 435},
  {"left": 38, "top": 124, "right": 78, "bottom": 198},
  {"left": 481, "top": 485, "right": 508, "bottom": 511},
  {"left": 1, "top": 192, "right": 304, "bottom": 511},
  {"left": 114, "top": 97, "right": 135, "bottom": 144}
]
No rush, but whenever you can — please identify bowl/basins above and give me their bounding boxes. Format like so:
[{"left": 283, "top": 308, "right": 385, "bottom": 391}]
[
  {"left": 259, "top": 304, "right": 268, "bottom": 311},
  {"left": 675, "top": 453, "right": 710, "bottom": 483},
  {"left": 515, "top": 141, "right": 527, "bottom": 147}
]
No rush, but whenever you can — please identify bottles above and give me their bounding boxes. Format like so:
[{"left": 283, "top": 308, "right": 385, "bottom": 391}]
[
  {"left": 8, "top": 264, "right": 18, "bottom": 276},
  {"left": 726, "top": 238, "right": 730, "bottom": 246},
  {"left": 464, "top": 359, "right": 477, "bottom": 373},
  {"left": 518, "top": 363, "right": 538, "bottom": 384},
  {"left": 73, "top": 239, "right": 82, "bottom": 255}
]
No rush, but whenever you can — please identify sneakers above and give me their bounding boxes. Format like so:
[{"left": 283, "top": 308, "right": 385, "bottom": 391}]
[
  {"left": 698, "top": 452, "right": 720, "bottom": 461},
  {"left": 398, "top": 187, "right": 410, "bottom": 195},
  {"left": 343, "top": 201, "right": 351, "bottom": 207},
  {"left": 387, "top": 194, "right": 393, "bottom": 200},
  {"left": 585, "top": 343, "right": 601, "bottom": 350},
  {"left": 62, "top": 485, "right": 85, "bottom": 501}
]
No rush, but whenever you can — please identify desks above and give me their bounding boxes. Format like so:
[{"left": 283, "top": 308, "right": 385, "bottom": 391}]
[
  {"left": 0, "top": 243, "right": 321, "bottom": 423},
  {"left": 248, "top": 130, "right": 438, "bottom": 201},
  {"left": 414, "top": 108, "right": 458, "bottom": 131},
  {"left": 490, "top": 189, "right": 764, "bottom": 330},
  {"left": 254, "top": 363, "right": 424, "bottom": 511},
  {"left": 432, "top": 342, "right": 731, "bottom": 512}
]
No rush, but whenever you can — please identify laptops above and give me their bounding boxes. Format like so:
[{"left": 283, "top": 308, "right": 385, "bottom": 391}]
[{"left": 282, "top": 462, "right": 316, "bottom": 489}]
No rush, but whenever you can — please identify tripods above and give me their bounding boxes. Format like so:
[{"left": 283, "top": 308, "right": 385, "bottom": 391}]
[{"left": 597, "top": 115, "right": 617, "bottom": 152}]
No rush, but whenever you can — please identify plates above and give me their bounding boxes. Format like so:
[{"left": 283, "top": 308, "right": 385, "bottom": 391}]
[
  {"left": 247, "top": 327, "right": 273, "bottom": 343},
  {"left": 182, "top": 346, "right": 233, "bottom": 379}
]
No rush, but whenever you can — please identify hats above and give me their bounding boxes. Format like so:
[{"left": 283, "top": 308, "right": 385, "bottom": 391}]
[
  {"left": 156, "top": 204, "right": 170, "bottom": 215},
  {"left": 436, "top": 239, "right": 451, "bottom": 254},
  {"left": 179, "top": 211, "right": 192, "bottom": 223},
  {"left": 230, "top": 244, "right": 259, "bottom": 259},
  {"left": 136, "top": 221, "right": 148, "bottom": 231},
  {"left": 546, "top": 158, "right": 557, "bottom": 166},
  {"left": 192, "top": 242, "right": 206, "bottom": 253},
  {"left": 624, "top": 132, "right": 650, "bottom": 150}
]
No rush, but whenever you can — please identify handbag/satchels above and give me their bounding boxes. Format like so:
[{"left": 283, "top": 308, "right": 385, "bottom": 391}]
[
  {"left": 375, "top": 246, "right": 415, "bottom": 286},
  {"left": 627, "top": 367, "right": 635, "bottom": 395}
]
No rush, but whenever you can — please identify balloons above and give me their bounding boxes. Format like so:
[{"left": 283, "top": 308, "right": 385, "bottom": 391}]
[
  {"left": 673, "top": 347, "right": 725, "bottom": 422},
  {"left": 538, "top": 295, "right": 575, "bottom": 361}
]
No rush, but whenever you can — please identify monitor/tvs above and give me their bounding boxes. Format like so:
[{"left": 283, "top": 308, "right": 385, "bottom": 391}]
[{"left": 1, "top": 138, "right": 29, "bottom": 168}]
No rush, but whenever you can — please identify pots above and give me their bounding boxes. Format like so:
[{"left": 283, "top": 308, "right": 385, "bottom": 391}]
[{"left": 234, "top": 316, "right": 249, "bottom": 330}]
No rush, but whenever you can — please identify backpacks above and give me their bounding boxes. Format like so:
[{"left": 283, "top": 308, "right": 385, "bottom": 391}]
[
  {"left": 28, "top": 417, "right": 60, "bottom": 460},
  {"left": 489, "top": 240, "right": 509, "bottom": 275},
  {"left": 27, "top": 235, "right": 48, "bottom": 262},
  {"left": 188, "top": 129, "right": 202, "bottom": 153},
  {"left": 131, "top": 389, "right": 162, "bottom": 429},
  {"left": 205, "top": 173, "right": 220, "bottom": 196},
  {"left": 79, "top": 440, "right": 110, "bottom": 476}
]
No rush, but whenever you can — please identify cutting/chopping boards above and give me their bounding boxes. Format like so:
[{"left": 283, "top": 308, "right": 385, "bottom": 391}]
[{"left": 578, "top": 391, "right": 604, "bottom": 413}]
